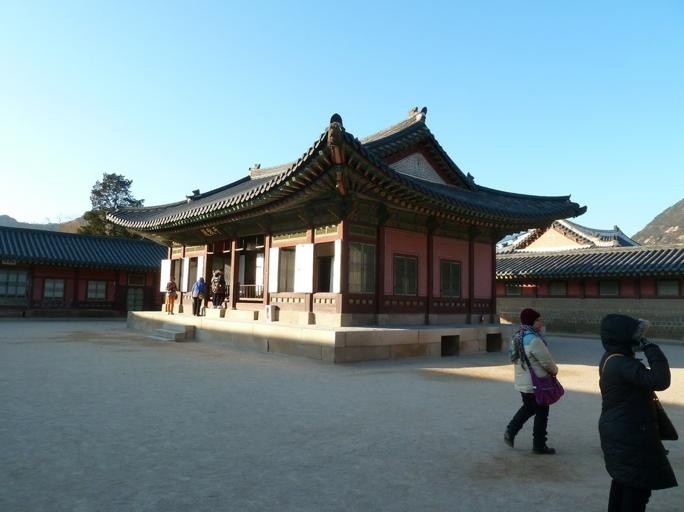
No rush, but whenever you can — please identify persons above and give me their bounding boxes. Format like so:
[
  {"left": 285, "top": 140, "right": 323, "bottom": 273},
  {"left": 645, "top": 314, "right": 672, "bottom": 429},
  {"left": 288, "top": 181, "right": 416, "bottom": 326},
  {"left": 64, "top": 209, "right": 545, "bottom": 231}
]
[
  {"left": 594, "top": 311, "right": 681, "bottom": 512},
  {"left": 503, "top": 307, "right": 557, "bottom": 455},
  {"left": 210, "top": 265, "right": 223, "bottom": 309},
  {"left": 191, "top": 276, "right": 205, "bottom": 316},
  {"left": 165, "top": 275, "right": 176, "bottom": 315}
]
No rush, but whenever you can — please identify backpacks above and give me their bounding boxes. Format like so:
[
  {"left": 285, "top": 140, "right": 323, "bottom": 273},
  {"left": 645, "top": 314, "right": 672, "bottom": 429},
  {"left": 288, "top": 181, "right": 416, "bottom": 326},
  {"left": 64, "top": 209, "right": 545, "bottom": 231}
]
[{"left": 211, "top": 278, "right": 225, "bottom": 294}]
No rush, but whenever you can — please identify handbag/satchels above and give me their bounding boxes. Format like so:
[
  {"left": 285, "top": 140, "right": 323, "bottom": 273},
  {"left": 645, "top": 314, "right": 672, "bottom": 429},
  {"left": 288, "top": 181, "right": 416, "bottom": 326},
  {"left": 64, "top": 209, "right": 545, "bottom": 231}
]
[
  {"left": 650, "top": 398, "right": 678, "bottom": 441},
  {"left": 531, "top": 375, "right": 564, "bottom": 405}
]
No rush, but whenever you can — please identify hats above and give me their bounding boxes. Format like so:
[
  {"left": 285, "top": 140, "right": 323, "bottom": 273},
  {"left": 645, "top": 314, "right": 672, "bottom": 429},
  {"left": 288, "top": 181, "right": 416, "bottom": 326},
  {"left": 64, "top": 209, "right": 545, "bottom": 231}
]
[{"left": 520, "top": 309, "right": 541, "bottom": 325}]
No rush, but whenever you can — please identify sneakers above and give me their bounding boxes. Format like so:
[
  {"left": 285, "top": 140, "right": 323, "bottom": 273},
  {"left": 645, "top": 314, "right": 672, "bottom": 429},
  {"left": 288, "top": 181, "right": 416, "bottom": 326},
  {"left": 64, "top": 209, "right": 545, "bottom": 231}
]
[
  {"left": 532, "top": 447, "right": 556, "bottom": 456},
  {"left": 503, "top": 432, "right": 515, "bottom": 445}
]
